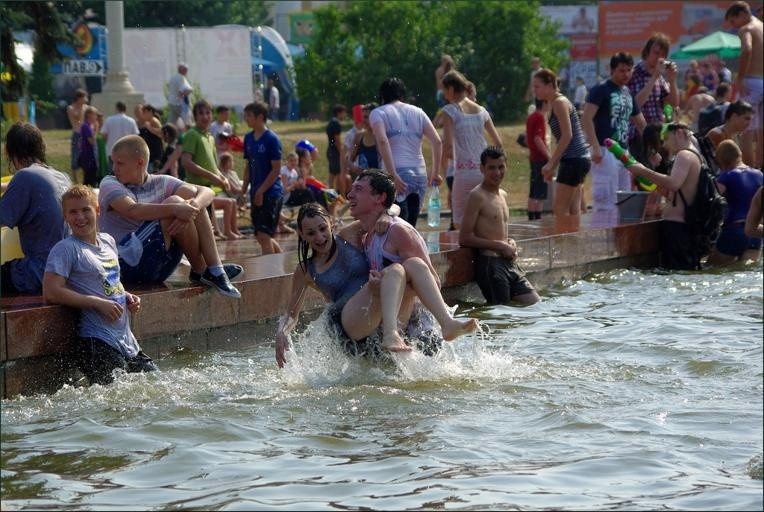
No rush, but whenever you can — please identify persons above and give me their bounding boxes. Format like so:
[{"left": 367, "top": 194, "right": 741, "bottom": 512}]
[
  {"left": 0, "top": 118, "right": 77, "bottom": 298},
  {"left": 40, "top": 184, "right": 160, "bottom": 386},
  {"left": 237, "top": 98, "right": 282, "bottom": 256},
  {"left": 366, "top": 56, "right": 502, "bottom": 230},
  {"left": 525, "top": 33, "right": 735, "bottom": 234},
  {"left": 275, "top": 201, "right": 480, "bottom": 368},
  {"left": 724, "top": 2, "right": 764, "bottom": 169},
  {"left": 281, "top": 102, "right": 385, "bottom": 212},
  {"left": 266, "top": 80, "right": 279, "bottom": 120},
  {"left": 458, "top": 145, "right": 541, "bottom": 309},
  {"left": 335, "top": 167, "right": 447, "bottom": 361},
  {"left": 706, "top": 140, "right": 763, "bottom": 267},
  {"left": 66, "top": 64, "right": 245, "bottom": 239},
  {"left": 630, "top": 121, "right": 713, "bottom": 273},
  {"left": 96, "top": 134, "right": 244, "bottom": 298}
]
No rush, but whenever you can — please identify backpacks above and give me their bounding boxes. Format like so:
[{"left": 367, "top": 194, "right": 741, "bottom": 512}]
[{"left": 676, "top": 149, "right": 728, "bottom": 257}]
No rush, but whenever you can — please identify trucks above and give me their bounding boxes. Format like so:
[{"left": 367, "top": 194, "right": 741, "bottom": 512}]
[{"left": 12, "top": 24, "right": 299, "bottom": 129}]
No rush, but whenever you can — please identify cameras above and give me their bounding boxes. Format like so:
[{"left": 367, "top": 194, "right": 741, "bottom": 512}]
[{"left": 660, "top": 61, "right": 673, "bottom": 72}]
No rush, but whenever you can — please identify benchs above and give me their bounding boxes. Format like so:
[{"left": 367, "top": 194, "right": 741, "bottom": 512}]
[{"left": 214, "top": 189, "right": 254, "bottom": 223}]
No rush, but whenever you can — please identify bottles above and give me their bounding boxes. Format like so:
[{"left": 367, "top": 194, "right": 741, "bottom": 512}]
[{"left": 427, "top": 182, "right": 440, "bottom": 230}]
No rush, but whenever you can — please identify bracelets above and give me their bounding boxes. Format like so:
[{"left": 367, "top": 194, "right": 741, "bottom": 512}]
[{"left": 383, "top": 208, "right": 395, "bottom": 218}]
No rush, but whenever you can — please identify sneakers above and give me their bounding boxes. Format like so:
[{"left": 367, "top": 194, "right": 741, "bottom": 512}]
[
  {"left": 189, "top": 263, "right": 244, "bottom": 284},
  {"left": 200, "top": 267, "right": 241, "bottom": 299}
]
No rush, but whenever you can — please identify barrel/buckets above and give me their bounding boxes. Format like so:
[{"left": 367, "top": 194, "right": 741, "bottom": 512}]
[{"left": 612, "top": 190, "right": 652, "bottom": 224}]
